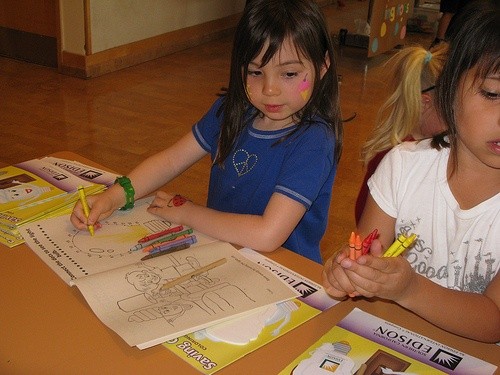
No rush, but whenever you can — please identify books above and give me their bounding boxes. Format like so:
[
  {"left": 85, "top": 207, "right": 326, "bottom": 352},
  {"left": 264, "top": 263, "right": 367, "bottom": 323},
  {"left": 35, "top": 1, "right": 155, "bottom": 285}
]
[
  {"left": 1, "top": 155, "right": 123, "bottom": 248},
  {"left": 20, "top": 191, "right": 348, "bottom": 375},
  {"left": 276, "top": 308, "right": 497, "bottom": 375}
]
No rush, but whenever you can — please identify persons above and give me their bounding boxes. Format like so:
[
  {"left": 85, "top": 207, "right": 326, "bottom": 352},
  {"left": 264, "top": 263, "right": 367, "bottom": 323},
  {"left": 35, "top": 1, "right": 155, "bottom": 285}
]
[
  {"left": 323, "top": 8, "right": 500, "bottom": 342},
  {"left": 70, "top": 0, "right": 357, "bottom": 265}
]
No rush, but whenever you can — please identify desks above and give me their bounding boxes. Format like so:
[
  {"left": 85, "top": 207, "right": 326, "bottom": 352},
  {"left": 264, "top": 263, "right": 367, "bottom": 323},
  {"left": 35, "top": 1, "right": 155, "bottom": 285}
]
[{"left": 0, "top": 151, "right": 500, "bottom": 375}]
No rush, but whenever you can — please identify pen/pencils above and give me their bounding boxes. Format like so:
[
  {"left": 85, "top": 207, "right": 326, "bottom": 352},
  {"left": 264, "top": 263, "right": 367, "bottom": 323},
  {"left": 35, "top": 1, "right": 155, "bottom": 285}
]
[{"left": 160, "top": 250, "right": 226, "bottom": 291}]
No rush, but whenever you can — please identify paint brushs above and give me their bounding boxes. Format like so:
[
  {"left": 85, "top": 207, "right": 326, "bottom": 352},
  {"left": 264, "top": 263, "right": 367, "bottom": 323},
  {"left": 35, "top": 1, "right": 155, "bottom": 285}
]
[
  {"left": 348, "top": 229, "right": 378, "bottom": 296},
  {"left": 383, "top": 233, "right": 416, "bottom": 257},
  {"left": 131, "top": 225, "right": 197, "bottom": 261},
  {"left": 78, "top": 186, "right": 95, "bottom": 237}
]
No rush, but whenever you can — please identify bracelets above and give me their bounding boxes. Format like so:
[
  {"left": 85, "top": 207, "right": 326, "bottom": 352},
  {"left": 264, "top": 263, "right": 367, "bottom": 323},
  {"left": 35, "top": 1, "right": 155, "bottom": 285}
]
[{"left": 114, "top": 176, "right": 135, "bottom": 211}]
